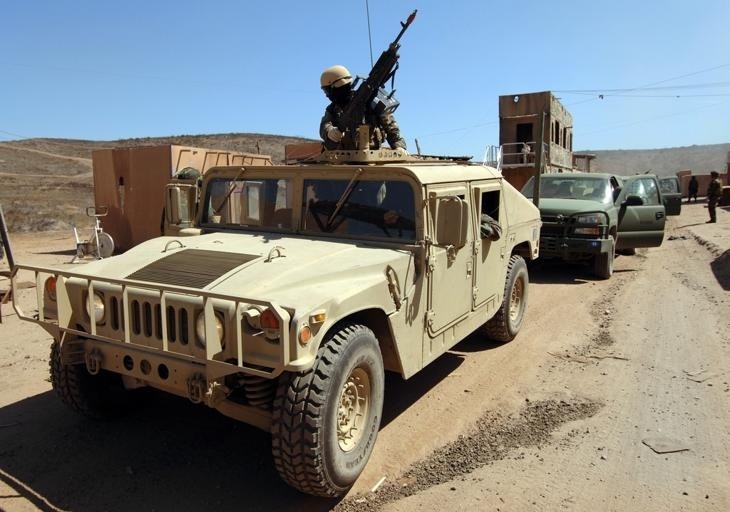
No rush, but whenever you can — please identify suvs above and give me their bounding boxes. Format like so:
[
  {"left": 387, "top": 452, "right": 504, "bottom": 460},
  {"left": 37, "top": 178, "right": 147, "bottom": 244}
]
[
  {"left": 621, "top": 175, "right": 681, "bottom": 217},
  {"left": 518, "top": 173, "right": 665, "bottom": 279}
]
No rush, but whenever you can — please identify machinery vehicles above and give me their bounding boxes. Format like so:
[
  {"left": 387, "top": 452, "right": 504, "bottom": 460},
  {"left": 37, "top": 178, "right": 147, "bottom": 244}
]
[{"left": 9, "top": 126, "right": 544, "bottom": 500}]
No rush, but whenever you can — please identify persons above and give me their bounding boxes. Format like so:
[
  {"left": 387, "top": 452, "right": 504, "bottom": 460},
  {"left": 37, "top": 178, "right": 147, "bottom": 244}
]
[
  {"left": 480, "top": 211, "right": 501, "bottom": 242},
  {"left": 160, "top": 166, "right": 213, "bottom": 236},
  {"left": 686, "top": 176, "right": 699, "bottom": 203},
  {"left": 320, "top": 66, "right": 409, "bottom": 157},
  {"left": 705, "top": 171, "right": 721, "bottom": 223}
]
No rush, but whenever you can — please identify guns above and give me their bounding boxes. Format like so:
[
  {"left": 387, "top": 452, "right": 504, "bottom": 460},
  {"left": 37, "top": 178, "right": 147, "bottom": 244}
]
[{"left": 322, "top": 9, "right": 417, "bottom": 150}]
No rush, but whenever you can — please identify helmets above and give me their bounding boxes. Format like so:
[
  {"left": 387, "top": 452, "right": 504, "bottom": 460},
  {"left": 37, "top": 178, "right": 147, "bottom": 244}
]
[{"left": 321, "top": 65, "right": 353, "bottom": 90}]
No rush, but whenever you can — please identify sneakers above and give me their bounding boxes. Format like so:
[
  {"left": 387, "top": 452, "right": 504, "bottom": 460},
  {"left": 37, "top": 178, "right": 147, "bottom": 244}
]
[{"left": 706, "top": 220, "right": 716, "bottom": 223}]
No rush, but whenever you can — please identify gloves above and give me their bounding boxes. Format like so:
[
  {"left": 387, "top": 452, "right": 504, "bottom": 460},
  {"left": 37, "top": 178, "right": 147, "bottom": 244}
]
[
  {"left": 393, "top": 147, "right": 410, "bottom": 157},
  {"left": 325, "top": 125, "right": 343, "bottom": 143}
]
